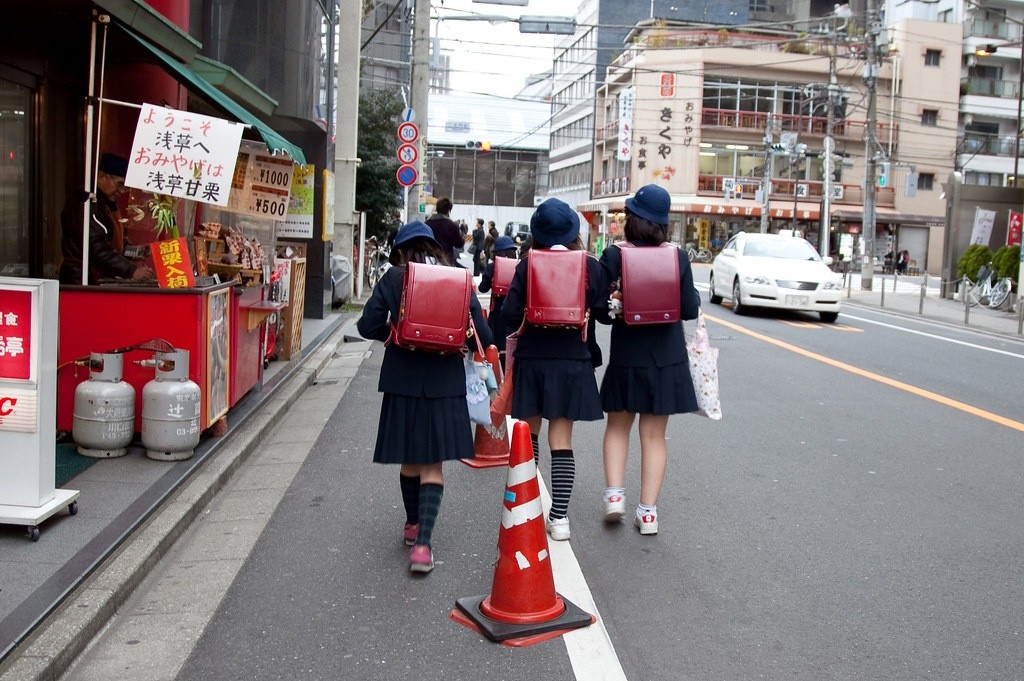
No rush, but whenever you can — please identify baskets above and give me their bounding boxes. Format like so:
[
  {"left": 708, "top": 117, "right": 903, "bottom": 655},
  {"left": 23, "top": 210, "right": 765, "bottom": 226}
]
[{"left": 975, "top": 265, "right": 997, "bottom": 283}]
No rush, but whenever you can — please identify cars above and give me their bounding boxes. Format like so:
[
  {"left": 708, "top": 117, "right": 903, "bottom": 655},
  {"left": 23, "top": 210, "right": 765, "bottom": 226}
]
[
  {"left": 505, "top": 221, "right": 532, "bottom": 243},
  {"left": 709, "top": 231, "right": 842, "bottom": 323}
]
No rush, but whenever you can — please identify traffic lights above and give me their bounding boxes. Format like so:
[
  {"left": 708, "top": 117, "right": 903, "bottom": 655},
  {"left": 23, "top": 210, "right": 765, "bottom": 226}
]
[
  {"left": 875, "top": 161, "right": 889, "bottom": 188},
  {"left": 465, "top": 138, "right": 491, "bottom": 151},
  {"left": 733, "top": 182, "right": 743, "bottom": 203}
]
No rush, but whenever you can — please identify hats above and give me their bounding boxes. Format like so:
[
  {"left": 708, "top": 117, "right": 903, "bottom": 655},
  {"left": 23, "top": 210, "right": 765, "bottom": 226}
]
[
  {"left": 626, "top": 184, "right": 671, "bottom": 225},
  {"left": 490, "top": 235, "right": 517, "bottom": 253},
  {"left": 387, "top": 219, "right": 444, "bottom": 267},
  {"left": 530, "top": 198, "right": 579, "bottom": 247}
]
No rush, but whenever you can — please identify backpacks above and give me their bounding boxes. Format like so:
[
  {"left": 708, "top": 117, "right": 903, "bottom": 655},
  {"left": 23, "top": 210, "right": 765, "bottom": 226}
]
[
  {"left": 612, "top": 241, "right": 682, "bottom": 325},
  {"left": 517, "top": 246, "right": 590, "bottom": 343},
  {"left": 382, "top": 261, "right": 472, "bottom": 355},
  {"left": 493, "top": 254, "right": 520, "bottom": 296}
]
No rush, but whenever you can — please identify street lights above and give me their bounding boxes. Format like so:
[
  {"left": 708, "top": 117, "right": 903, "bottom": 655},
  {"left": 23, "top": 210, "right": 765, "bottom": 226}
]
[{"left": 976, "top": 39, "right": 1024, "bottom": 187}]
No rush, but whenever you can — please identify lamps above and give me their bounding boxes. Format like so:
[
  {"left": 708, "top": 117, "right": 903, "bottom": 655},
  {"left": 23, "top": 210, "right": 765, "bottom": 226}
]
[{"left": 427, "top": 151, "right": 446, "bottom": 157}]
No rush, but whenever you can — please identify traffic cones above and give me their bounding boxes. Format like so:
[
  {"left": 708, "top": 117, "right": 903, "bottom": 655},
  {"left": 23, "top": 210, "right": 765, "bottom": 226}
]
[
  {"left": 450, "top": 421, "right": 597, "bottom": 647},
  {"left": 459, "top": 282, "right": 510, "bottom": 468}
]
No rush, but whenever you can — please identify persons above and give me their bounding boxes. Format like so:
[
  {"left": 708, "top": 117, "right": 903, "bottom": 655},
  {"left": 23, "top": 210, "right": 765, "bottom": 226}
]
[
  {"left": 451, "top": 218, "right": 517, "bottom": 329},
  {"left": 595, "top": 184, "right": 701, "bottom": 535},
  {"left": 58, "top": 152, "right": 155, "bottom": 285},
  {"left": 501, "top": 198, "right": 605, "bottom": 541},
  {"left": 357, "top": 220, "right": 492, "bottom": 571},
  {"left": 422, "top": 197, "right": 465, "bottom": 267}
]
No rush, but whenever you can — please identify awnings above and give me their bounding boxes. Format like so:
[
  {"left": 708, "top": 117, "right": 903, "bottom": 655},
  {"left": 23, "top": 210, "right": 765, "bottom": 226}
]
[{"left": 91, "top": 0, "right": 336, "bottom": 172}]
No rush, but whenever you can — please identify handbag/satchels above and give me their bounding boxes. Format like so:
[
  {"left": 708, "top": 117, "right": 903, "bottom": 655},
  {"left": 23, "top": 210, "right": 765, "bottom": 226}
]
[
  {"left": 685, "top": 306, "right": 723, "bottom": 420},
  {"left": 486, "top": 332, "right": 514, "bottom": 415},
  {"left": 461, "top": 349, "right": 493, "bottom": 427},
  {"left": 471, "top": 313, "right": 497, "bottom": 392},
  {"left": 468, "top": 244, "right": 476, "bottom": 254}
]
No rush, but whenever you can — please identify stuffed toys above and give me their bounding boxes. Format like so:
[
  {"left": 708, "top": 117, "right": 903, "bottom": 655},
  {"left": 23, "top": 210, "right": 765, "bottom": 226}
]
[{"left": 607, "top": 289, "right": 623, "bottom": 319}]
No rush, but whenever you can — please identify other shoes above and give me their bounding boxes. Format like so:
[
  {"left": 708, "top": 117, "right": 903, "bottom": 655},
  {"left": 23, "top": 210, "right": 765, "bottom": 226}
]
[
  {"left": 546, "top": 515, "right": 570, "bottom": 540},
  {"left": 409, "top": 543, "right": 435, "bottom": 573},
  {"left": 603, "top": 492, "right": 626, "bottom": 522},
  {"left": 634, "top": 509, "right": 659, "bottom": 535},
  {"left": 404, "top": 521, "right": 419, "bottom": 546}
]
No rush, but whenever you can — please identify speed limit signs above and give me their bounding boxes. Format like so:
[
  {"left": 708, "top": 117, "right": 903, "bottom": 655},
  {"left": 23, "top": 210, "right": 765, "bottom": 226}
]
[{"left": 397, "top": 121, "right": 419, "bottom": 143}]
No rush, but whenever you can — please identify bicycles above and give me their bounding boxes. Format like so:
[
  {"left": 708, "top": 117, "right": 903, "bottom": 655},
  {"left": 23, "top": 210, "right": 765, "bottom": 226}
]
[
  {"left": 367, "top": 230, "right": 392, "bottom": 288},
  {"left": 685, "top": 242, "right": 713, "bottom": 263},
  {"left": 964, "top": 261, "right": 1012, "bottom": 308}
]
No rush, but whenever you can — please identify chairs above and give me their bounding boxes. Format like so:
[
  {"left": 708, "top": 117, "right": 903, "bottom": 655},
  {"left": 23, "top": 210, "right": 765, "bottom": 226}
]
[
  {"left": 908, "top": 259, "right": 919, "bottom": 276},
  {"left": 882, "top": 260, "right": 897, "bottom": 274}
]
